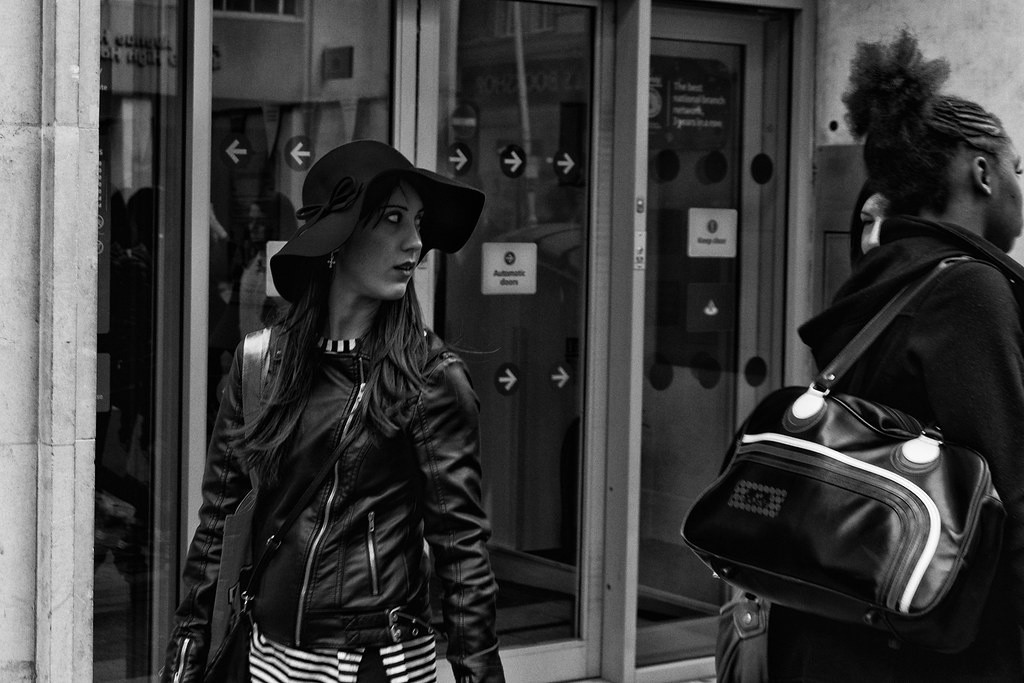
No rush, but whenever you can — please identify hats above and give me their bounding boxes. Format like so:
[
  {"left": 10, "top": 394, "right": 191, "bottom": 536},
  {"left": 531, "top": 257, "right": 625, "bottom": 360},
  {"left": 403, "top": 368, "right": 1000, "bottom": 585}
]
[{"left": 270, "top": 139, "right": 486, "bottom": 309}]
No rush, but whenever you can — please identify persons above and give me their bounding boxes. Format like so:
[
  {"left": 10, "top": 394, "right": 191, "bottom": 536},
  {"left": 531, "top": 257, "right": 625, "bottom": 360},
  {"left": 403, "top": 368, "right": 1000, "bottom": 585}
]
[
  {"left": 112, "top": 169, "right": 297, "bottom": 598},
  {"left": 765, "top": 31, "right": 1024, "bottom": 683},
  {"left": 160, "top": 140, "right": 505, "bottom": 683}
]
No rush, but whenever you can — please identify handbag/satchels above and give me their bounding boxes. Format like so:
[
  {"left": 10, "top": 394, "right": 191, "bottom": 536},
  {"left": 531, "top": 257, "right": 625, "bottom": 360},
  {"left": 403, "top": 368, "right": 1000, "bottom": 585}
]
[
  {"left": 714, "top": 587, "right": 770, "bottom": 683},
  {"left": 208, "top": 325, "right": 271, "bottom": 683},
  {"left": 679, "top": 259, "right": 1007, "bottom": 653}
]
